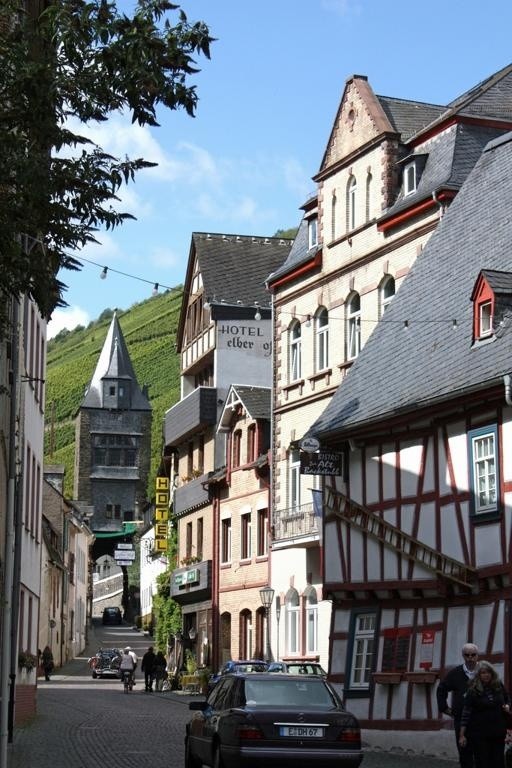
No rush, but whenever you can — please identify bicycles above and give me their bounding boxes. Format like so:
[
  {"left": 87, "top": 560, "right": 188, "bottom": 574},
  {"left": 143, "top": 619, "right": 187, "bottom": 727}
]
[{"left": 123, "top": 671, "right": 135, "bottom": 692}]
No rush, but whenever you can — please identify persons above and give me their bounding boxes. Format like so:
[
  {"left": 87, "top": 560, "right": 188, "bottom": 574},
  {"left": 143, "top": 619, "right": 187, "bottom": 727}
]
[
  {"left": 141, "top": 646, "right": 155, "bottom": 693},
  {"left": 151, "top": 651, "right": 167, "bottom": 692},
  {"left": 457, "top": 660, "right": 511, "bottom": 767},
  {"left": 41, "top": 646, "right": 54, "bottom": 682},
  {"left": 117, "top": 644, "right": 139, "bottom": 686},
  {"left": 436, "top": 642, "right": 479, "bottom": 768}
]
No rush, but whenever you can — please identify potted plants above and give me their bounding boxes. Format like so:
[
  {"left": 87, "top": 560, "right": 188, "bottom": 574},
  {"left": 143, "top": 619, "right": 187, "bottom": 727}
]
[{"left": 199, "top": 669, "right": 212, "bottom": 695}]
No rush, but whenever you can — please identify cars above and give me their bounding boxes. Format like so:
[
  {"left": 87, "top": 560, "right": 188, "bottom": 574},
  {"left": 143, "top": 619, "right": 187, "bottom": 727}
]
[
  {"left": 100, "top": 606, "right": 122, "bottom": 624},
  {"left": 92, "top": 647, "right": 122, "bottom": 677},
  {"left": 184, "top": 659, "right": 364, "bottom": 767}
]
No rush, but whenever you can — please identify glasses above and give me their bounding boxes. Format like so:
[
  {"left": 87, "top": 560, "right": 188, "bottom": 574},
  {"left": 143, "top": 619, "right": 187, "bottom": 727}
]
[{"left": 464, "top": 654, "right": 476, "bottom": 657}]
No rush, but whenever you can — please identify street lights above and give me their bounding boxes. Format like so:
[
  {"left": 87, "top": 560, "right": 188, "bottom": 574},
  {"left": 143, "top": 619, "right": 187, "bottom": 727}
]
[{"left": 259, "top": 583, "right": 274, "bottom": 663}]
